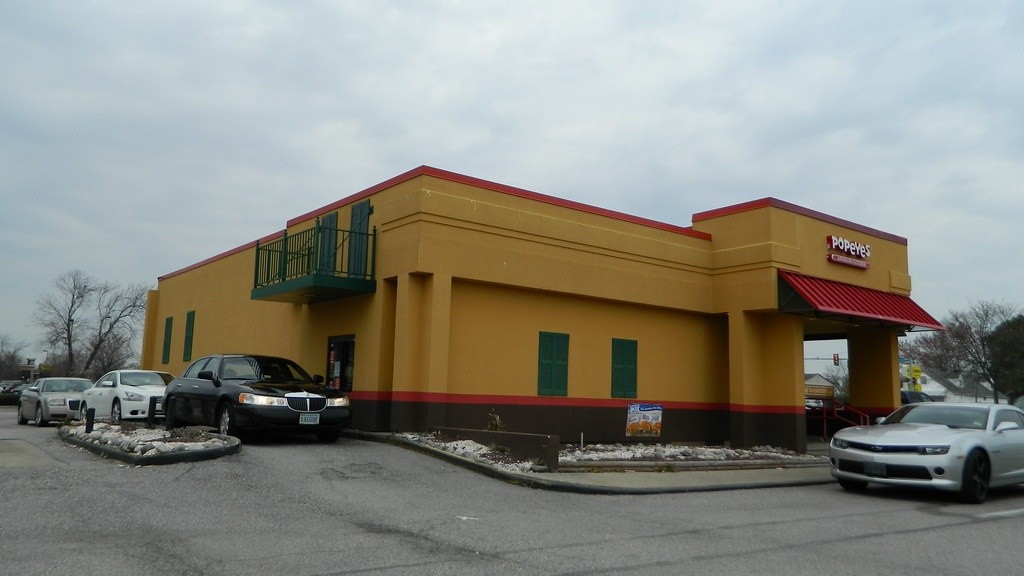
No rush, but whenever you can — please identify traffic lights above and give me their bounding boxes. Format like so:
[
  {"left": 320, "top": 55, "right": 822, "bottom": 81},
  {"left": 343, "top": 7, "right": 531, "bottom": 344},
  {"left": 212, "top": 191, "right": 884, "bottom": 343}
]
[{"left": 834, "top": 354, "right": 838, "bottom": 366}]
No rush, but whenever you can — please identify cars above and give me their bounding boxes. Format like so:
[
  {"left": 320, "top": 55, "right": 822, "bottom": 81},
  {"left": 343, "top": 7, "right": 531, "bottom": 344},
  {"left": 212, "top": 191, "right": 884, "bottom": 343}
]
[
  {"left": 829, "top": 402, "right": 1024, "bottom": 502},
  {"left": 79, "top": 368, "right": 180, "bottom": 424},
  {"left": 1, "top": 379, "right": 26, "bottom": 393},
  {"left": 18, "top": 377, "right": 95, "bottom": 429},
  {"left": 161, "top": 352, "right": 352, "bottom": 446}
]
